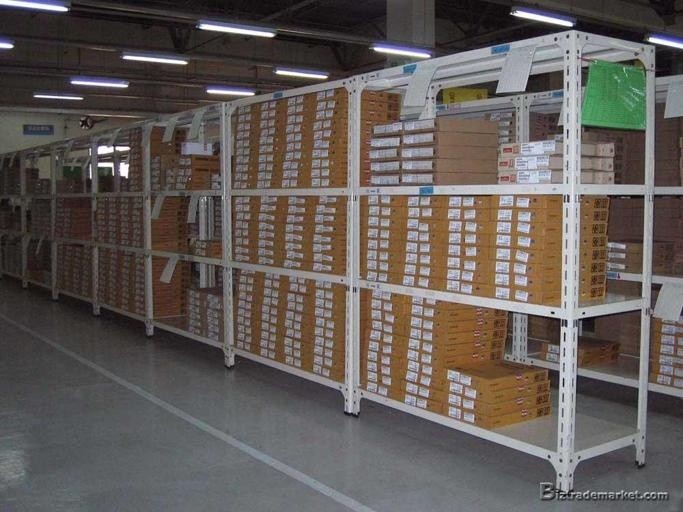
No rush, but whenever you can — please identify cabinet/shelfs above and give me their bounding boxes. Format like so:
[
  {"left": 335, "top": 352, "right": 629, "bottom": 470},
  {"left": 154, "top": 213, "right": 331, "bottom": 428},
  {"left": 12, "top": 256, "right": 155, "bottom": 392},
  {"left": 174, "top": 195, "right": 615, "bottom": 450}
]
[{"left": 1, "top": 31, "right": 682, "bottom": 495}]
